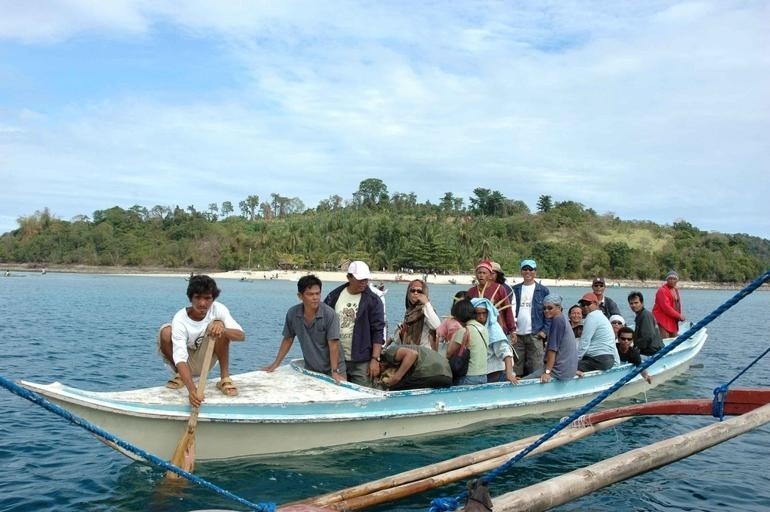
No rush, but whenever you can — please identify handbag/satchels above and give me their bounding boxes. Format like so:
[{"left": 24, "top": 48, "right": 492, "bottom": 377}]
[{"left": 449, "top": 327, "right": 471, "bottom": 376}]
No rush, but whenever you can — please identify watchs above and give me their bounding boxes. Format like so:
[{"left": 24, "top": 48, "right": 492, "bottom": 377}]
[
  {"left": 371, "top": 356, "right": 381, "bottom": 362},
  {"left": 331, "top": 368, "right": 340, "bottom": 373},
  {"left": 543, "top": 368, "right": 552, "bottom": 375}
]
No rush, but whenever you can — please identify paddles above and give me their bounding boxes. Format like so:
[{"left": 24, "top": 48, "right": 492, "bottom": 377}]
[{"left": 163, "top": 334, "right": 218, "bottom": 487}]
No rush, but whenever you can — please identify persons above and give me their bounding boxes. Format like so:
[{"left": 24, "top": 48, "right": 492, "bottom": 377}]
[
  {"left": 368, "top": 281, "right": 390, "bottom": 352},
  {"left": 522, "top": 292, "right": 586, "bottom": 385},
  {"left": 627, "top": 292, "right": 665, "bottom": 357},
  {"left": 614, "top": 326, "right": 653, "bottom": 387},
  {"left": 651, "top": 271, "right": 686, "bottom": 339},
  {"left": 259, "top": 273, "right": 347, "bottom": 383},
  {"left": 470, "top": 297, "right": 520, "bottom": 388},
  {"left": 157, "top": 271, "right": 246, "bottom": 397},
  {"left": 452, "top": 289, "right": 467, "bottom": 306},
  {"left": 568, "top": 304, "right": 585, "bottom": 327},
  {"left": 445, "top": 299, "right": 490, "bottom": 388},
  {"left": 434, "top": 290, "right": 472, "bottom": 354},
  {"left": 609, "top": 313, "right": 626, "bottom": 336},
  {"left": 40, "top": 266, "right": 49, "bottom": 278},
  {"left": 322, "top": 260, "right": 385, "bottom": 388},
  {"left": 3, "top": 268, "right": 10, "bottom": 277},
  {"left": 576, "top": 290, "right": 623, "bottom": 372},
  {"left": 510, "top": 258, "right": 553, "bottom": 377},
  {"left": 381, "top": 278, "right": 442, "bottom": 352},
  {"left": 489, "top": 260, "right": 520, "bottom": 330},
  {"left": 571, "top": 323, "right": 584, "bottom": 337},
  {"left": 379, "top": 338, "right": 453, "bottom": 389},
  {"left": 576, "top": 276, "right": 622, "bottom": 319},
  {"left": 465, "top": 260, "right": 519, "bottom": 348}
]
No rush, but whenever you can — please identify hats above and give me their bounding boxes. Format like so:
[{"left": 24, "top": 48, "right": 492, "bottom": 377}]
[
  {"left": 571, "top": 319, "right": 585, "bottom": 328},
  {"left": 609, "top": 314, "right": 625, "bottom": 326},
  {"left": 520, "top": 259, "right": 537, "bottom": 269},
  {"left": 577, "top": 292, "right": 598, "bottom": 307},
  {"left": 475, "top": 261, "right": 492, "bottom": 273},
  {"left": 665, "top": 271, "right": 679, "bottom": 281},
  {"left": 348, "top": 261, "right": 370, "bottom": 281},
  {"left": 489, "top": 262, "right": 505, "bottom": 275},
  {"left": 592, "top": 275, "right": 605, "bottom": 287},
  {"left": 542, "top": 293, "right": 563, "bottom": 304}
]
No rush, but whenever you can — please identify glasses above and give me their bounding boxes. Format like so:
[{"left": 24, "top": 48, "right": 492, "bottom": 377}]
[
  {"left": 522, "top": 268, "right": 533, "bottom": 271},
  {"left": 612, "top": 322, "right": 621, "bottom": 325},
  {"left": 543, "top": 306, "right": 553, "bottom": 310},
  {"left": 620, "top": 337, "right": 632, "bottom": 342},
  {"left": 409, "top": 287, "right": 422, "bottom": 294},
  {"left": 581, "top": 302, "right": 592, "bottom": 307},
  {"left": 593, "top": 284, "right": 604, "bottom": 288}
]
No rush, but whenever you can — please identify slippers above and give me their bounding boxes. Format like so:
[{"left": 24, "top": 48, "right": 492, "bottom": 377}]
[
  {"left": 165, "top": 372, "right": 185, "bottom": 389},
  {"left": 217, "top": 377, "right": 239, "bottom": 398}
]
